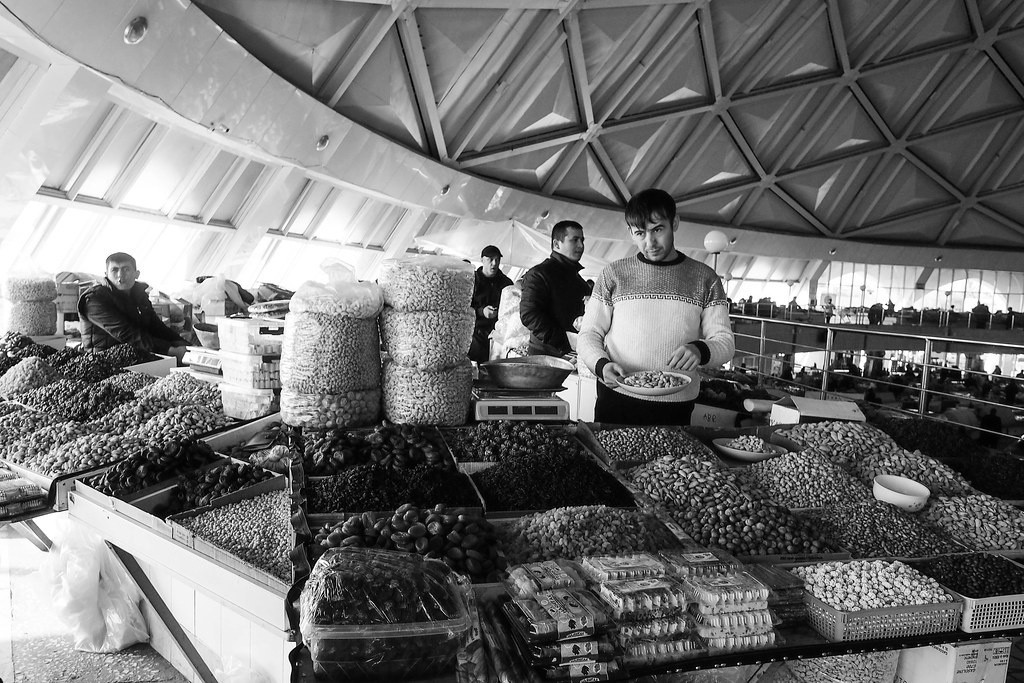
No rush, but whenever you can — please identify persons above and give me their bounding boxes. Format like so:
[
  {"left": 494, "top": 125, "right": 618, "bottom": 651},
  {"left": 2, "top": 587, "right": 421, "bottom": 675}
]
[
  {"left": 1016, "top": 370, "right": 1024, "bottom": 385},
  {"left": 77, "top": 252, "right": 192, "bottom": 367},
  {"left": 972, "top": 304, "right": 1021, "bottom": 329},
  {"left": 992, "top": 365, "right": 1002, "bottom": 375},
  {"left": 726, "top": 295, "right": 802, "bottom": 313},
  {"left": 885, "top": 300, "right": 895, "bottom": 311},
  {"left": 576, "top": 188, "right": 734, "bottom": 425},
  {"left": 519, "top": 221, "right": 593, "bottom": 358},
  {"left": 467, "top": 245, "right": 514, "bottom": 367}
]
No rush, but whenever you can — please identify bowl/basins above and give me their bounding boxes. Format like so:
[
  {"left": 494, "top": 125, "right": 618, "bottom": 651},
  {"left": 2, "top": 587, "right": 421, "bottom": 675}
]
[
  {"left": 193, "top": 322, "right": 220, "bottom": 350},
  {"left": 480, "top": 354, "right": 576, "bottom": 389},
  {"left": 872, "top": 474, "right": 931, "bottom": 513}
]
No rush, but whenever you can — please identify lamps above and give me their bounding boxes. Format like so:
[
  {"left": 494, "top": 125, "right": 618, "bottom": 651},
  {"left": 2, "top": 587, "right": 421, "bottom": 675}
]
[
  {"left": 860, "top": 285, "right": 866, "bottom": 306},
  {"left": 724, "top": 272, "right": 732, "bottom": 294},
  {"left": 787, "top": 280, "right": 794, "bottom": 299},
  {"left": 703, "top": 231, "right": 728, "bottom": 272},
  {"left": 944, "top": 291, "right": 950, "bottom": 308}
]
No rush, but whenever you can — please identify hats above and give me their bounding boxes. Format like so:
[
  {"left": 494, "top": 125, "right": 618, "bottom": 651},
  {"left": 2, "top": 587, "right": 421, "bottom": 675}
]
[{"left": 481, "top": 245, "right": 503, "bottom": 258}]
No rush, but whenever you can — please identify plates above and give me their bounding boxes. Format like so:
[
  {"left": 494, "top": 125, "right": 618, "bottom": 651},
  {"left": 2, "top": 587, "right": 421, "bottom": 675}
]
[
  {"left": 712, "top": 438, "right": 788, "bottom": 463},
  {"left": 615, "top": 370, "right": 692, "bottom": 396}
]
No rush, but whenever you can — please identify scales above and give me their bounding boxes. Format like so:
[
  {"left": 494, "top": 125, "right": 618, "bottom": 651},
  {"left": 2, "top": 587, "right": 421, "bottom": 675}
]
[
  {"left": 470, "top": 385, "right": 569, "bottom": 421},
  {"left": 181, "top": 346, "right": 222, "bottom": 375}
]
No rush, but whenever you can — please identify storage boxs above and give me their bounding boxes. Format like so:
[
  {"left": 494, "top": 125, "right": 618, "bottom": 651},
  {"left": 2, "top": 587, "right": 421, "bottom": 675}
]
[{"left": 0, "top": 300, "right": 1024, "bottom": 683}]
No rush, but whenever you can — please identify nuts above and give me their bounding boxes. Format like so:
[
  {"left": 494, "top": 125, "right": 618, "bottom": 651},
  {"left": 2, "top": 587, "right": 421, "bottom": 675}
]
[
  {"left": 279, "top": 264, "right": 476, "bottom": 431},
  {"left": 3, "top": 279, "right": 59, "bottom": 335},
  {"left": 0, "top": 331, "right": 295, "bottom": 586},
  {"left": 625, "top": 370, "right": 688, "bottom": 389},
  {"left": 311, "top": 417, "right": 1024, "bottom": 683}
]
